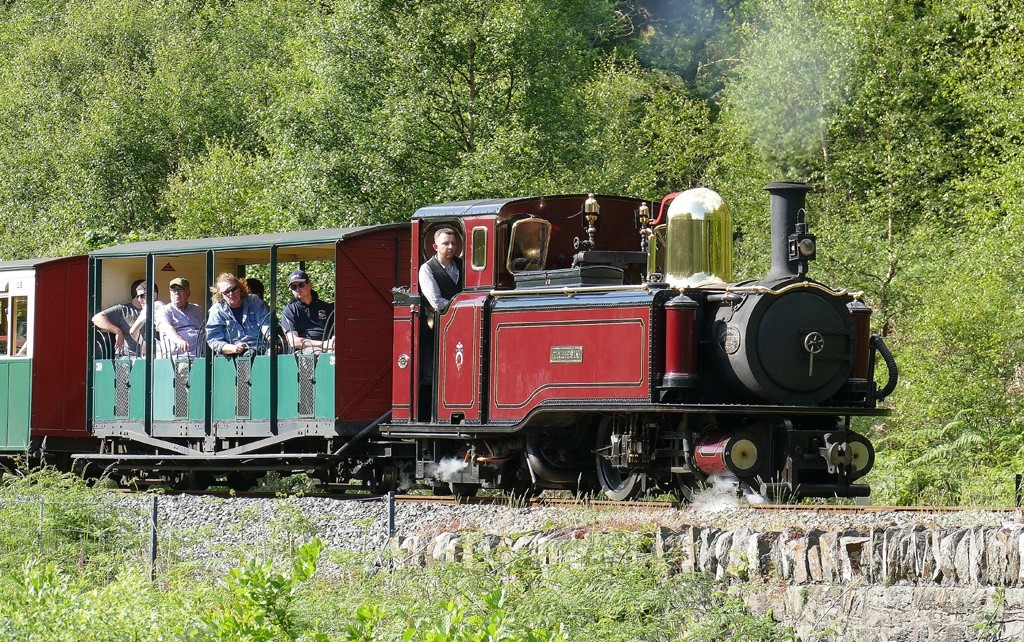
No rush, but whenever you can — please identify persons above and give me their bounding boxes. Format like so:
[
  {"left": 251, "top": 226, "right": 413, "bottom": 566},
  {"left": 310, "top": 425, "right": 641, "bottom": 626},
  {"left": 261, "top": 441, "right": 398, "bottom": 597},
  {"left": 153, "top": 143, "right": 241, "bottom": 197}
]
[
  {"left": 246, "top": 278, "right": 270, "bottom": 313},
  {"left": 418, "top": 228, "right": 462, "bottom": 335},
  {"left": 154, "top": 278, "right": 204, "bottom": 372},
  {"left": 205, "top": 272, "right": 281, "bottom": 355},
  {"left": 91, "top": 279, "right": 146, "bottom": 374},
  {"left": 281, "top": 270, "right": 334, "bottom": 354},
  {"left": 129, "top": 280, "right": 167, "bottom": 359}
]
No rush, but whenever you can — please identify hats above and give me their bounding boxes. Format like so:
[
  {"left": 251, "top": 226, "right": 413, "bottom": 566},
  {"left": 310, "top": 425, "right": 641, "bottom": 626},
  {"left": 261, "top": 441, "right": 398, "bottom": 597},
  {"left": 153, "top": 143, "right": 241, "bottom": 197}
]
[
  {"left": 169, "top": 277, "right": 189, "bottom": 289},
  {"left": 288, "top": 271, "right": 309, "bottom": 286}
]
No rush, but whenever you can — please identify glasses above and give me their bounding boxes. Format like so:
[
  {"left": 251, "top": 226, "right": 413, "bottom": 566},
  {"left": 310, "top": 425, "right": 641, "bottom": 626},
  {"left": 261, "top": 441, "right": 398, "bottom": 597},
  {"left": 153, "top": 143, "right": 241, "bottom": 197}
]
[
  {"left": 137, "top": 293, "right": 145, "bottom": 298},
  {"left": 291, "top": 282, "right": 307, "bottom": 290},
  {"left": 221, "top": 285, "right": 237, "bottom": 295}
]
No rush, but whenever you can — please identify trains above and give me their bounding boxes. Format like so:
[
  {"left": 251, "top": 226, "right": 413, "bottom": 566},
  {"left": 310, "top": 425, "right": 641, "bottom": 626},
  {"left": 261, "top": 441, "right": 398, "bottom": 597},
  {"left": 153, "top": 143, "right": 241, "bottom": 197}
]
[{"left": 0, "top": 181, "right": 900, "bottom": 512}]
